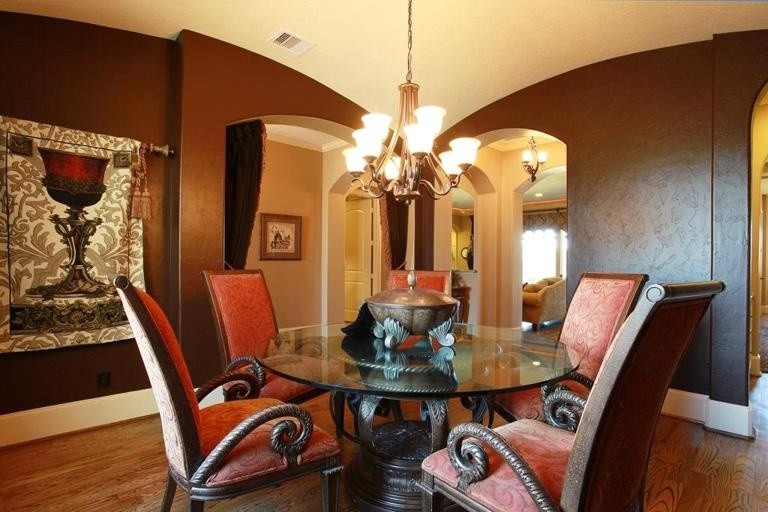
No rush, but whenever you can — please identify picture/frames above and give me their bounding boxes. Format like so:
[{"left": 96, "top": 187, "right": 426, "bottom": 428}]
[{"left": 260, "top": 214, "right": 302, "bottom": 261}]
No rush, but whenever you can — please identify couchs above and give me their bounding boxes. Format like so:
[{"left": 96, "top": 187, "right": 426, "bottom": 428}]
[{"left": 522, "top": 280, "right": 567, "bottom": 330}]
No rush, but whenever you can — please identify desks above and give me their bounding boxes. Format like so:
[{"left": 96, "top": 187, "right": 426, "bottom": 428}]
[{"left": 254, "top": 325, "right": 580, "bottom": 512}]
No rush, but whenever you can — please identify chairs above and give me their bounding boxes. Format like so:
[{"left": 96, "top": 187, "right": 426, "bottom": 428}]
[
  {"left": 113, "top": 275, "right": 344, "bottom": 512},
  {"left": 201, "top": 268, "right": 345, "bottom": 440},
  {"left": 388, "top": 270, "right": 451, "bottom": 294},
  {"left": 421, "top": 281, "right": 725, "bottom": 512},
  {"left": 489, "top": 271, "right": 649, "bottom": 431}
]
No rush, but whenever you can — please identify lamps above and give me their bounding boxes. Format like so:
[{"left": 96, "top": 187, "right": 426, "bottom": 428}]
[
  {"left": 342, "top": 0, "right": 481, "bottom": 205},
  {"left": 520, "top": 134, "right": 547, "bottom": 182}
]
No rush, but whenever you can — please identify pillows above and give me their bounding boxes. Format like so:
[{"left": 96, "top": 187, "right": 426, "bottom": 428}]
[{"left": 523, "top": 277, "right": 562, "bottom": 293}]
